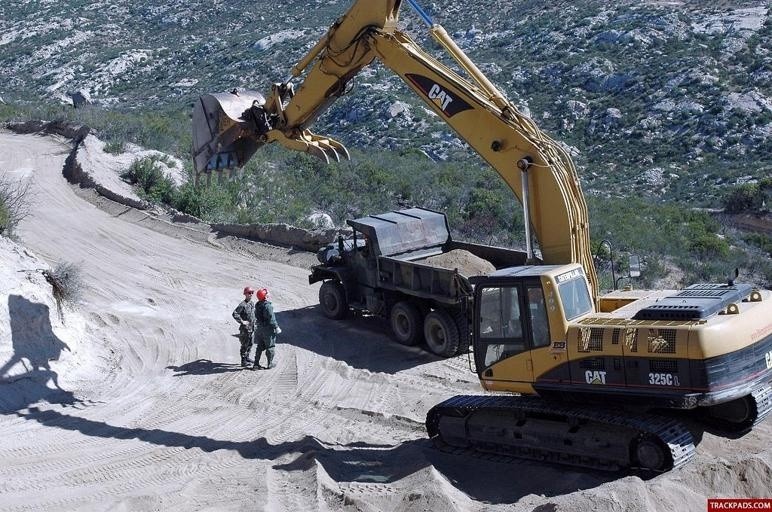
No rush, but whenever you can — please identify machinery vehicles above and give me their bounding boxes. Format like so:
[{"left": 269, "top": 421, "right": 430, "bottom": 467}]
[{"left": 188, "top": 1, "right": 771, "bottom": 475}]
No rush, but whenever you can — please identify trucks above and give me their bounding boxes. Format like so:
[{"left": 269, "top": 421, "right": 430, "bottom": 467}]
[{"left": 308, "top": 205, "right": 536, "bottom": 358}]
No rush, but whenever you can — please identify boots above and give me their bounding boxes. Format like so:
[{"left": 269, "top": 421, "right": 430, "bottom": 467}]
[
  {"left": 246, "top": 355, "right": 255, "bottom": 363},
  {"left": 267, "top": 360, "right": 276, "bottom": 368},
  {"left": 253, "top": 361, "right": 265, "bottom": 370},
  {"left": 241, "top": 357, "right": 253, "bottom": 366}
]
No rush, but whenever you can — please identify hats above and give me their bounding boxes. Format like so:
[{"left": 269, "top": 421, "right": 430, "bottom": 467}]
[
  {"left": 243, "top": 287, "right": 255, "bottom": 295},
  {"left": 256, "top": 288, "right": 268, "bottom": 300}
]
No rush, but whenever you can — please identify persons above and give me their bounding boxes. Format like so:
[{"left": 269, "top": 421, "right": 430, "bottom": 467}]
[
  {"left": 253, "top": 289, "right": 281, "bottom": 371},
  {"left": 232, "top": 287, "right": 255, "bottom": 366}
]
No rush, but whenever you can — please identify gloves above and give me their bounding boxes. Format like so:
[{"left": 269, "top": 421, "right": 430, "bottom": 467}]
[
  {"left": 275, "top": 326, "right": 282, "bottom": 334},
  {"left": 241, "top": 319, "right": 250, "bottom": 326}
]
